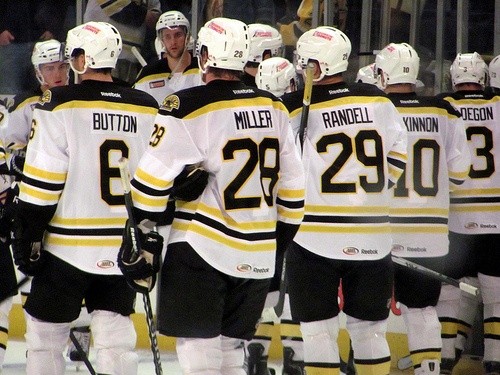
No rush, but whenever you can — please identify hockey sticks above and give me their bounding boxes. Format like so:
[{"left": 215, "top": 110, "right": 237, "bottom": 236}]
[
  {"left": 392, "top": 256, "right": 478, "bottom": 295},
  {"left": 116, "top": 156, "right": 164, "bottom": 375},
  {"left": 130, "top": 45, "right": 147, "bottom": 67},
  {"left": 259, "top": 57, "right": 317, "bottom": 322}
]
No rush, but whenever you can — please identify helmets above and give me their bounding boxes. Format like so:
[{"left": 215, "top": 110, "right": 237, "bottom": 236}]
[
  {"left": 193, "top": 16, "right": 251, "bottom": 74},
  {"left": 293, "top": 25, "right": 352, "bottom": 83},
  {"left": 245, "top": 23, "right": 284, "bottom": 64},
  {"left": 30, "top": 39, "right": 65, "bottom": 85},
  {"left": 153, "top": 9, "right": 195, "bottom": 61},
  {"left": 254, "top": 57, "right": 298, "bottom": 97},
  {"left": 487, "top": 54, "right": 500, "bottom": 89},
  {"left": 63, "top": 20, "right": 123, "bottom": 75},
  {"left": 448, "top": 52, "right": 489, "bottom": 89},
  {"left": 355, "top": 63, "right": 386, "bottom": 93},
  {"left": 374, "top": 41, "right": 421, "bottom": 86}
]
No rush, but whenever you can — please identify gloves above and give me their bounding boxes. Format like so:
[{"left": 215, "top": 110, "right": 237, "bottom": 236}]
[
  {"left": 11, "top": 238, "right": 32, "bottom": 265},
  {"left": 168, "top": 163, "right": 209, "bottom": 203},
  {"left": 116, "top": 233, "right": 154, "bottom": 282}
]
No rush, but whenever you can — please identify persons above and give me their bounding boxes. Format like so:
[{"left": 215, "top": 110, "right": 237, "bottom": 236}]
[
  {"left": 83, "top": 0, "right": 492, "bottom": 102},
  {"left": 1, "top": 0, "right": 423, "bottom": 362},
  {"left": 11, "top": 23, "right": 159, "bottom": 375},
  {"left": 370, "top": 43, "right": 471, "bottom": 375},
  {"left": 436, "top": 52, "right": 500, "bottom": 375},
  {"left": 118, "top": 17, "right": 304, "bottom": 375},
  {"left": 489, "top": 55, "right": 500, "bottom": 96},
  {"left": 279, "top": 26, "right": 406, "bottom": 375}
]
